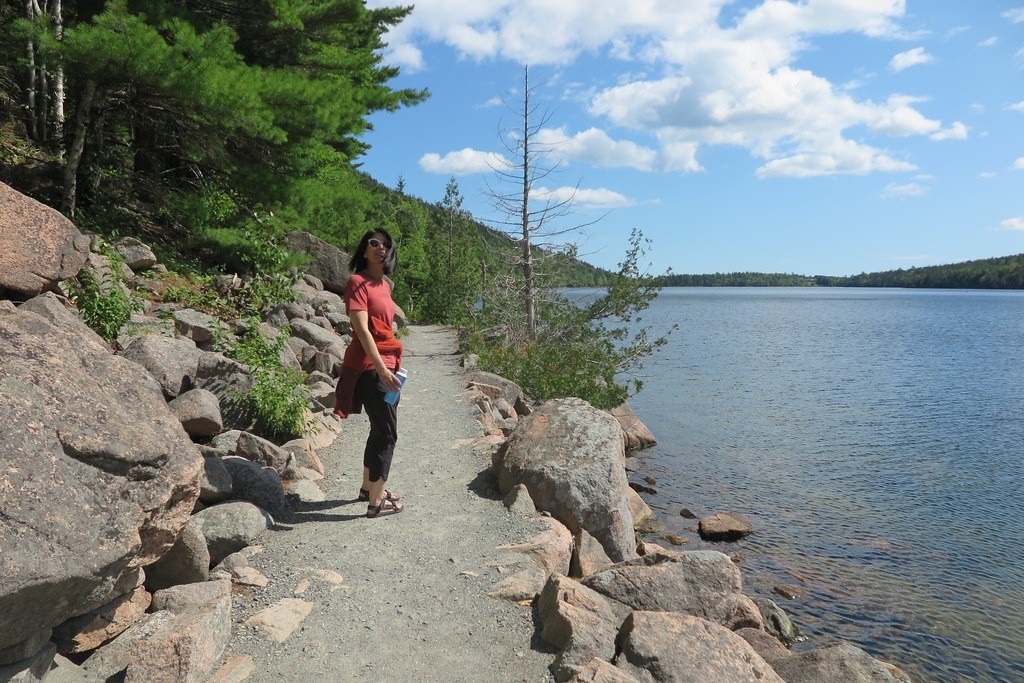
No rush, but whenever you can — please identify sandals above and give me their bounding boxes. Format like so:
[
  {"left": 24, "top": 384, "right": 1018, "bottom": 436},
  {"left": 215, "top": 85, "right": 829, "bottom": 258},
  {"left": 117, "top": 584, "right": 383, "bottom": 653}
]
[
  {"left": 366, "top": 497, "right": 402, "bottom": 518},
  {"left": 358, "top": 486, "right": 399, "bottom": 501}
]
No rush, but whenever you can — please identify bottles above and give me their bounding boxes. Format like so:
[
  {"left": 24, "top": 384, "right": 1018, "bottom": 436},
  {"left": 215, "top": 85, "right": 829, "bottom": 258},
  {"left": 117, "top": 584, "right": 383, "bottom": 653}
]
[{"left": 384, "top": 368, "right": 408, "bottom": 405}]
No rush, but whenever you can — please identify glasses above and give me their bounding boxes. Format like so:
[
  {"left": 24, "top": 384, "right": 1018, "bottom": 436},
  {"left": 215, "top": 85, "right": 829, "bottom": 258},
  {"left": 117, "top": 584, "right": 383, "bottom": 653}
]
[{"left": 368, "top": 238, "right": 391, "bottom": 250}]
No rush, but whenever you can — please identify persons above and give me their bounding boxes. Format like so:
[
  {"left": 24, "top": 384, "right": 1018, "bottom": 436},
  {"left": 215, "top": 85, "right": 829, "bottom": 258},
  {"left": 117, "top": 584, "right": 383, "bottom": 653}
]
[{"left": 333, "top": 228, "right": 404, "bottom": 518}]
